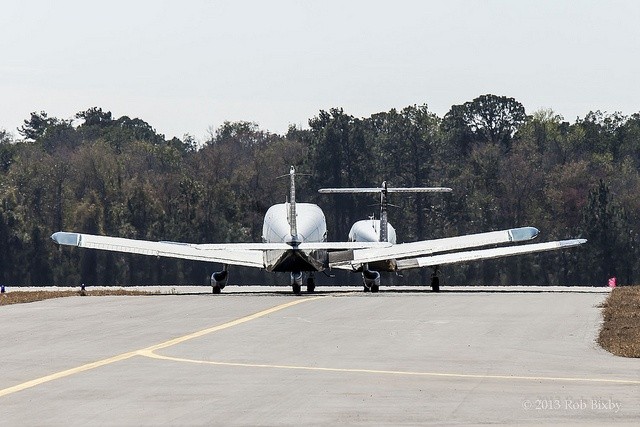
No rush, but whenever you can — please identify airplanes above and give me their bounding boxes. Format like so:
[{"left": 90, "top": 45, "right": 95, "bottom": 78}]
[
  {"left": 316, "top": 180, "right": 588, "bottom": 293},
  {"left": 49, "top": 165, "right": 542, "bottom": 297}
]
